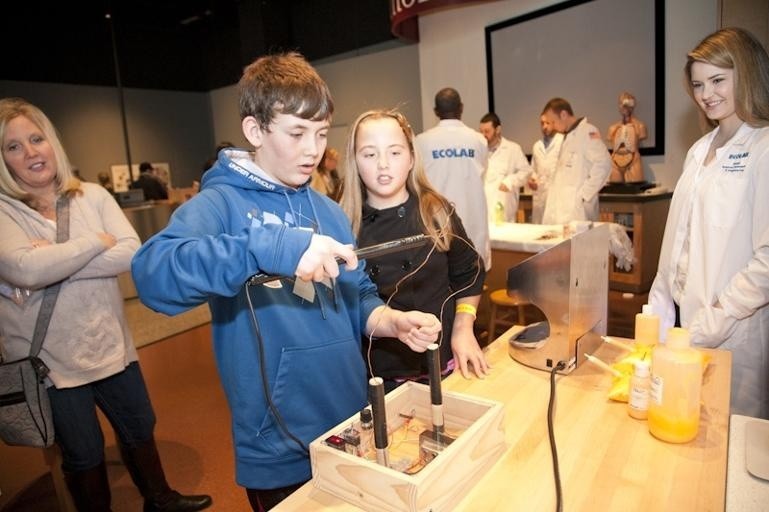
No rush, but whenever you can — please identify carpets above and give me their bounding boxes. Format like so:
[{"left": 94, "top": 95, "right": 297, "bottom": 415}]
[{"left": 125, "top": 294, "right": 215, "bottom": 353}]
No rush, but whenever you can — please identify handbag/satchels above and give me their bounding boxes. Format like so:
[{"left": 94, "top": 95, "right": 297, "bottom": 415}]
[{"left": 0, "top": 357, "right": 56, "bottom": 450}]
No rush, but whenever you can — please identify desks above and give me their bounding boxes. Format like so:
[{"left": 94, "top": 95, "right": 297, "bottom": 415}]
[
  {"left": 726, "top": 412, "right": 769, "bottom": 512},
  {"left": 476, "top": 219, "right": 611, "bottom": 346},
  {"left": 269, "top": 324, "right": 734, "bottom": 512}
]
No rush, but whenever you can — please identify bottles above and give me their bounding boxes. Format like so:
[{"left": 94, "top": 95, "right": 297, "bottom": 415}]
[
  {"left": 634, "top": 302, "right": 661, "bottom": 348},
  {"left": 496, "top": 200, "right": 504, "bottom": 226},
  {"left": 645, "top": 327, "right": 705, "bottom": 445},
  {"left": 628, "top": 361, "right": 653, "bottom": 420}
]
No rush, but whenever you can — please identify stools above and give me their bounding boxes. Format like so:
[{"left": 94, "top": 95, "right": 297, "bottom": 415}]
[{"left": 487, "top": 289, "right": 531, "bottom": 346}]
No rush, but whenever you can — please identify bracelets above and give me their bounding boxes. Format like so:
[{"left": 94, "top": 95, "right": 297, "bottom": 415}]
[{"left": 456, "top": 303, "right": 477, "bottom": 320}]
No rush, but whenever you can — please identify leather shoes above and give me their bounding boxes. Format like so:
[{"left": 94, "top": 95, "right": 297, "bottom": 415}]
[{"left": 142, "top": 492, "right": 212, "bottom": 512}]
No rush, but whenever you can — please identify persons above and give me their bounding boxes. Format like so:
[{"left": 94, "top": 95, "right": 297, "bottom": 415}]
[
  {"left": 412, "top": 87, "right": 491, "bottom": 274},
  {"left": 127, "top": 50, "right": 442, "bottom": 512},
  {"left": 647, "top": 27, "right": 769, "bottom": 420},
  {"left": 332, "top": 97, "right": 492, "bottom": 396},
  {"left": 528, "top": 97, "right": 612, "bottom": 225},
  {"left": 604, "top": 92, "right": 647, "bottom": 184},
  {"left": 0, "top": 96, "right": 211, "bottom": 512},
  {"left": 128, "top": 164, "right": 171, "bottom": 200},
  {"left": 205, "top": 141, "right": 236, "bottom": 171},
  {"left": 480, "top": 112, "right": 532, "bottom": 223}
]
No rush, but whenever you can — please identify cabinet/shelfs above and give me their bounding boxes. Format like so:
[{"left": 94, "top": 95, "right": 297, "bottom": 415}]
[{"left": 516, "top": 192, "right": 672, "bottom": 294}]
